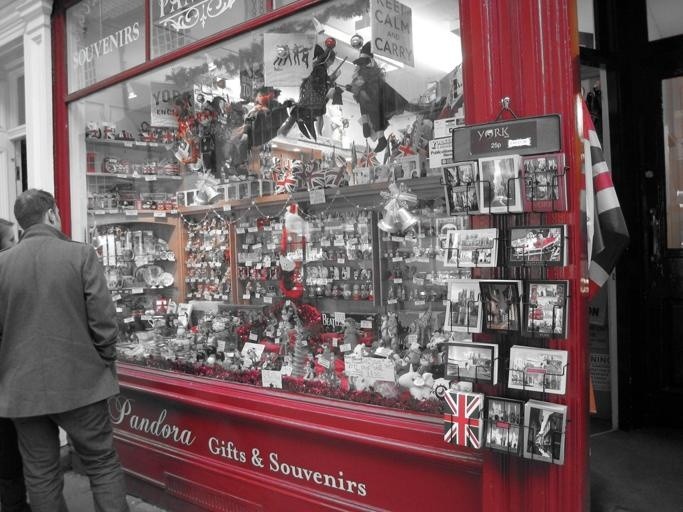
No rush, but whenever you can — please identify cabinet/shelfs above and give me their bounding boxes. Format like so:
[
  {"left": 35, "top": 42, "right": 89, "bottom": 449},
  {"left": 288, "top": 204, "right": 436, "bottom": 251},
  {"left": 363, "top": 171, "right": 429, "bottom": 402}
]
[{"left": 84, "top": 135, "right": 470, "bottom": 382}]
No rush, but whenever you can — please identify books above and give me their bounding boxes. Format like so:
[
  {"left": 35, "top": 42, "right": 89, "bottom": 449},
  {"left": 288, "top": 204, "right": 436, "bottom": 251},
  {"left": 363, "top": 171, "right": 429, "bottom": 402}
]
[{"left": 436, "top": 150, "right": 570, "bottom": 467}]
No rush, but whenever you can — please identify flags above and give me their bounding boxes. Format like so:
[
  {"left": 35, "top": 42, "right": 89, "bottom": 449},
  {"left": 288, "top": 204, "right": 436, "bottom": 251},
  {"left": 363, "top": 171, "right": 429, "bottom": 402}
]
[{"left": 575, "top": 94, "right": 632, "bottom": 303}]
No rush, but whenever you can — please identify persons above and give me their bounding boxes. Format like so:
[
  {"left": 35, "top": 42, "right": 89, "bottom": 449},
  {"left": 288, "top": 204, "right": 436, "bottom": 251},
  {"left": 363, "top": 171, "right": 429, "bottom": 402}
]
[
  {"left": 0, "top": 190, "right": 131, "bottom": 511},
  {"left": 0, "top": 220, "right": 34, "bottom": 512}
]
